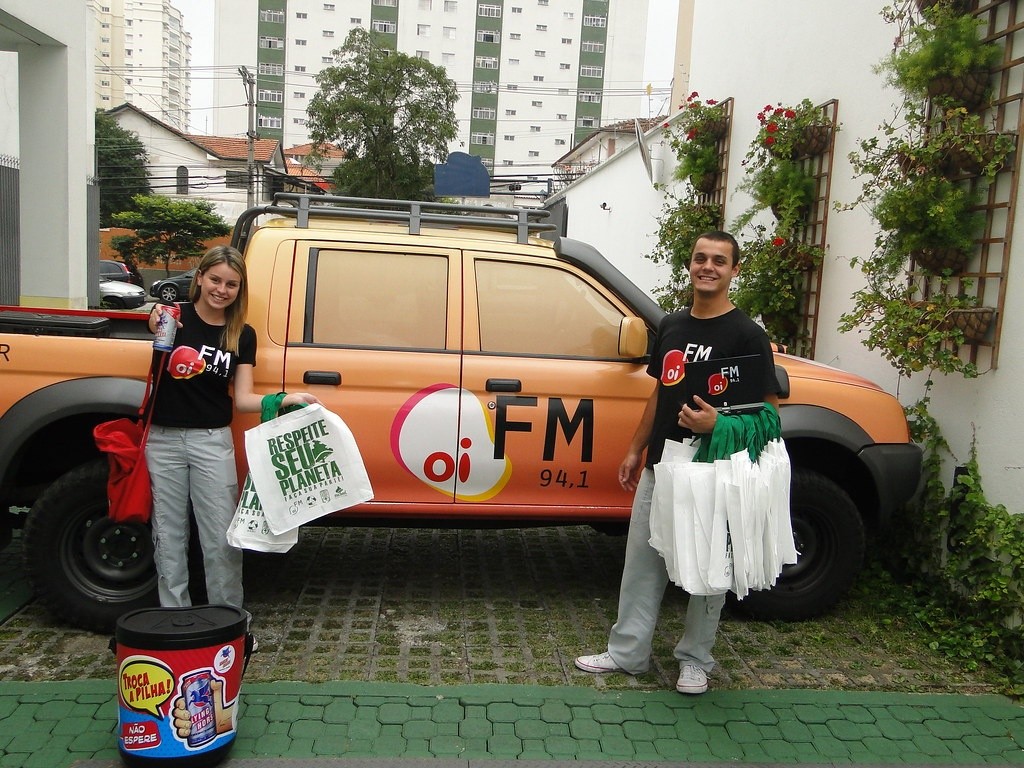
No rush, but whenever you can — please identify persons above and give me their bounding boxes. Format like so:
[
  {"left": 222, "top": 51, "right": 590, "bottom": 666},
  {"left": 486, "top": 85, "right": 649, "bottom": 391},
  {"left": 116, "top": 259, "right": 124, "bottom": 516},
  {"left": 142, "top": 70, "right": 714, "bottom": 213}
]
[
  {"left": 574, "top": 230, "right": 782, "bottom": 694},
  {"left": 143, "top": 246, "right": 326, "bottom": 652}
]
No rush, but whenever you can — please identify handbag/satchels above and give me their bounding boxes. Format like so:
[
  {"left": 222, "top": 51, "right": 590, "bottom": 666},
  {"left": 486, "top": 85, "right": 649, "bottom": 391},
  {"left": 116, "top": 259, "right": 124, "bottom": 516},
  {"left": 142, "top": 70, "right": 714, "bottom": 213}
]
[
  {"left": 225, "top": 392, "right": 374, "bottom": 553},
  {"left": 647, "top": 402, "right": 802, "bottom": 601},
  {"left": 93, "top": 418, "right": 152, "bottom": 524}
]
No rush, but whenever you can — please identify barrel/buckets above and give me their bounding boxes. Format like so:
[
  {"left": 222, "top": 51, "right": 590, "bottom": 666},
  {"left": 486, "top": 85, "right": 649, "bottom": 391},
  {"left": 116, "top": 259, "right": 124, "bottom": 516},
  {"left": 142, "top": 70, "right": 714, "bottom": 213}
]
[{"left": 108, "top": 604, "right": 254, "bottom": 768}]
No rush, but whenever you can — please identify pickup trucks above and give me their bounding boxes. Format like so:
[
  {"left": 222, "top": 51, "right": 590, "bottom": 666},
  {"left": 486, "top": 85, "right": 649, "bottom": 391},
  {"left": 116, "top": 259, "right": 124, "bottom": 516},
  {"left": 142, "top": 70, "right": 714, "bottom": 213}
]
[{"left": 0, "top": 192, "right": 923, "bottom": 634}]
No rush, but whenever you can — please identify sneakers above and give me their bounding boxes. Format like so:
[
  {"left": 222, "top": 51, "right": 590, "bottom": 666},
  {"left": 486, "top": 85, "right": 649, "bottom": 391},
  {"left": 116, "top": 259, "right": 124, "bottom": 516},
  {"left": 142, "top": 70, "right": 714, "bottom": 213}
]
[
  {"left": 676, "top": 665, "right": 710, "bottom": 693},
  {"left": 575, "top": 651, "right": 626, "bottom": 672}
]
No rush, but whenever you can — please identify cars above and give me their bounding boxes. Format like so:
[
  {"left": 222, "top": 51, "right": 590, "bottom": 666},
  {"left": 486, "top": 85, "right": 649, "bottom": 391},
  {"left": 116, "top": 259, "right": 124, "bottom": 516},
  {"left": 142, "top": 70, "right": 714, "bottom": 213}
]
[
  {"left": 99, "top": 275, "right": 148, "bottom": 310},
  {"left": 150, "top": 268, "right": 198, "bottom": 302},
  {"left": 99, "top": 260, "right": 146, "bottom": 292}
]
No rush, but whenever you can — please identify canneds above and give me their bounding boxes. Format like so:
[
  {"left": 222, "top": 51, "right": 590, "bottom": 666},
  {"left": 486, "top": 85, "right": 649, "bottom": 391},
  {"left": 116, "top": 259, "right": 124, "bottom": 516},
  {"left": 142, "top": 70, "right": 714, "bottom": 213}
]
[{"left": 153, "top": 306, "right": 181, "bottom": 352}]
